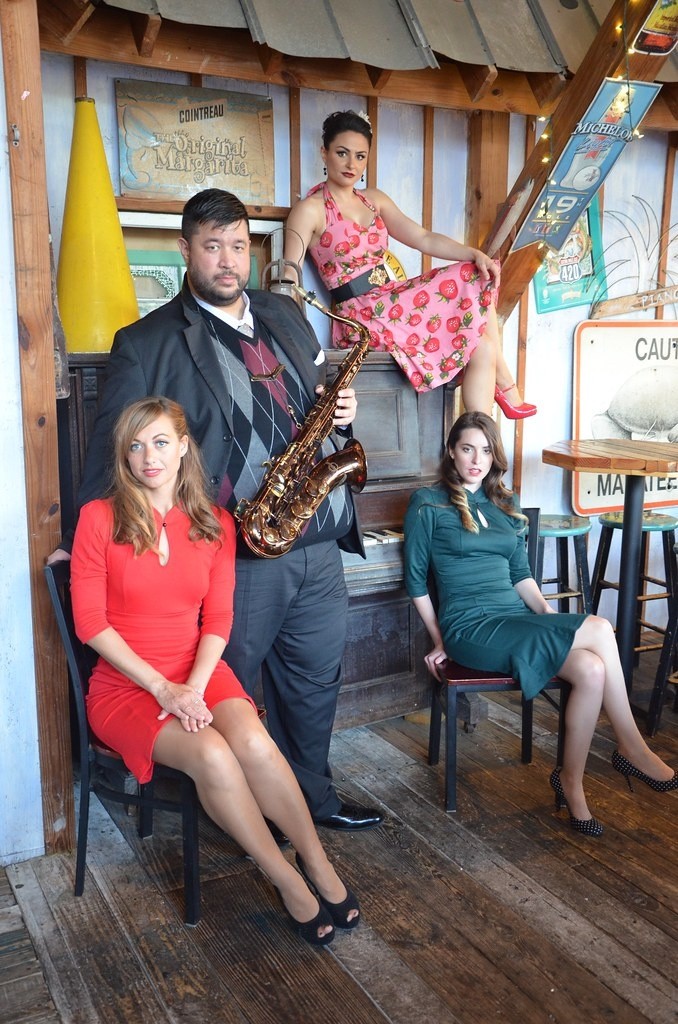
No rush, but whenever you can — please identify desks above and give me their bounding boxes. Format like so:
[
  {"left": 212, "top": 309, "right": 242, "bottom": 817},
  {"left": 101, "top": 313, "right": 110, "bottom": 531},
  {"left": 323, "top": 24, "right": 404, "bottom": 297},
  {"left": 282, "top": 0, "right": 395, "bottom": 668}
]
[{"left": 543, "top": 440, "right": 678, "bottom": 698}]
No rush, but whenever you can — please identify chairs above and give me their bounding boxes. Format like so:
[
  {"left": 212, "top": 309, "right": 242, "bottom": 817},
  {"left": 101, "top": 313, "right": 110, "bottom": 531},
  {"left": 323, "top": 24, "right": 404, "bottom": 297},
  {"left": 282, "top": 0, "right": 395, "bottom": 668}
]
[
  {"left": 427, "top": 508, "right": 572, "bottom": 813},
  {"left": 43, "top": 558, "right": 266, "bottom": 928}
]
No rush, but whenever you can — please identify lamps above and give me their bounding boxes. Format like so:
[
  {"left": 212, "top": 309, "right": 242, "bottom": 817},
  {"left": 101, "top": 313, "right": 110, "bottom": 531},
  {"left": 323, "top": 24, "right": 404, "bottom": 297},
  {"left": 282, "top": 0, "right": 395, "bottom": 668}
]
[{"left": 260, "top": 228, "right": 307, "bottom": 316}]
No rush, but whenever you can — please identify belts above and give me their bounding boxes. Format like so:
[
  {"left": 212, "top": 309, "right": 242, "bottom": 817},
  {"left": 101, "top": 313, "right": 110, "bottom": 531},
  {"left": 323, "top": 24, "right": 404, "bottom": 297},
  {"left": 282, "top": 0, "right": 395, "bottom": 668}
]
[{"left": 329, "top": 263, "right": 391, "bottom": 304}]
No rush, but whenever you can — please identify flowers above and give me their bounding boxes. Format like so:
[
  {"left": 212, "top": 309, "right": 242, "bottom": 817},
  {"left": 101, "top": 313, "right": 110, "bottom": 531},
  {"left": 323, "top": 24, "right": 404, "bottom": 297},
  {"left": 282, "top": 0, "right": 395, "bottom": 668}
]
[{"left": 359, "top": 110, "right": 373, "bottom": 135}]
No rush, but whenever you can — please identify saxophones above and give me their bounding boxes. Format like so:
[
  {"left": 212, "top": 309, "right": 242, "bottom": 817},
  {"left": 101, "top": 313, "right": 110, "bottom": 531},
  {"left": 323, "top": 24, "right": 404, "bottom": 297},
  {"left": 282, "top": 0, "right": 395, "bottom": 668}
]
[{"left": 232, "top": 282, "right": 373, "bottom": 560}]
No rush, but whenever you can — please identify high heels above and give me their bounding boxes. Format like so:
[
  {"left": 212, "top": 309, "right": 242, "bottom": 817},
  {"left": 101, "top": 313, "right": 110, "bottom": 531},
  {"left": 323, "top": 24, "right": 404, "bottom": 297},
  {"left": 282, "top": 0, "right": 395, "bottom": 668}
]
[
  {"left": 296, "top": 852, "right": 360, "bottom": 929},
  {"left": 273, "top": 885, "right": 335, "bottom": 945},
  {"left": 611, "top": 750, "right": 678, "bottom": 792},
  {"left": 550, "top": 768, "right": 604, "bottom": 837},
  {"left": 491, "top": 382, "right": 537, "bottom": 419}
]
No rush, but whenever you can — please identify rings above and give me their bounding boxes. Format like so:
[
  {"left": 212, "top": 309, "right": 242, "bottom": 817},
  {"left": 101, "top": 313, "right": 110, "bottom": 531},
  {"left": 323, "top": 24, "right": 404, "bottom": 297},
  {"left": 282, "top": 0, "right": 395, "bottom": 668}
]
[{"left": 195, "top": 700, "right": 199, "bottom": 703}]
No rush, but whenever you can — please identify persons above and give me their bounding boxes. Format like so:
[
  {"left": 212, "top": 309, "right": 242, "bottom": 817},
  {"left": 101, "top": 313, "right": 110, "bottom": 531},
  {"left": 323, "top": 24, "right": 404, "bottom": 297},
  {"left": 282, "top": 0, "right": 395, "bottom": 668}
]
[
  {"left": 284, "top": 111, "right": 536, "bottom": 419},
  {"left": 70, "top": 397, "right": 360, "bottom": 944},
  {"left": 46, "top": 188, "right": 385, "bottom": 852},
  {"left": 404, "top": 412, "right": 678, "bottom": 834}
]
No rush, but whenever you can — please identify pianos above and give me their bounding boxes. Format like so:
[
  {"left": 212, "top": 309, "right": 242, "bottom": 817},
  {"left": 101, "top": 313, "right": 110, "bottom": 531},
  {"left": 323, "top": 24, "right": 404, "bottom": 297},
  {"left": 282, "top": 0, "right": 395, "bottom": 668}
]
[{"left": 58, "top": 348, "right": 483, "bottom": 813}]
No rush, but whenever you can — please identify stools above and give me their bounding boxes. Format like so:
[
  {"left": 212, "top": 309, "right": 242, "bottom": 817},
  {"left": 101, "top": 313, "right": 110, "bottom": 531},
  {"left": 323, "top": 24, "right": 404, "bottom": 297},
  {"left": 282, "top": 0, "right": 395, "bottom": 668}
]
[
  {"left": 591, "top": 511, "right": 678, "bottom": 711},
  {"left": 524, "top": 514, "right": 593, "bottom": 616}
]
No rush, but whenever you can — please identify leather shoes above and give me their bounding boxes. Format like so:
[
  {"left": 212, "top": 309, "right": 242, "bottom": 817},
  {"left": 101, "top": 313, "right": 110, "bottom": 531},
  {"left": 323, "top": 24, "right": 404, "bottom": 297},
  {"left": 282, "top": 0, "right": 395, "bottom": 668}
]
[
  {"left": 313, "top": 803, "right": 385, "bottom": 831},
  {"left": 268, "top": 821, "right": 291, "bottom": 848}
]
